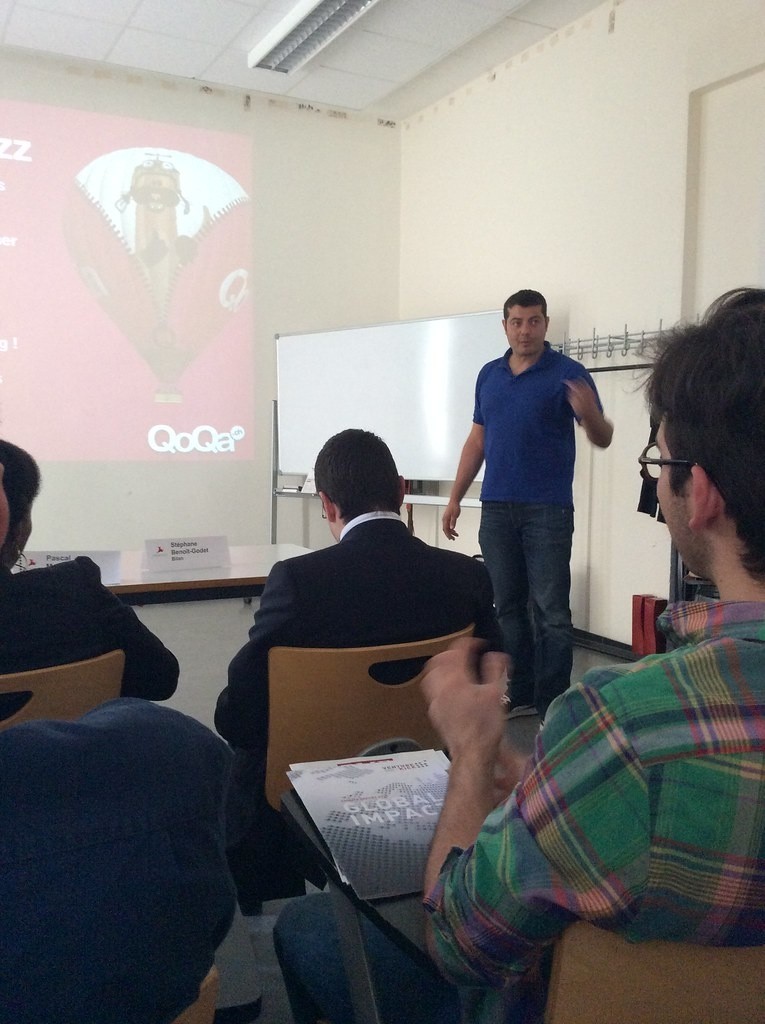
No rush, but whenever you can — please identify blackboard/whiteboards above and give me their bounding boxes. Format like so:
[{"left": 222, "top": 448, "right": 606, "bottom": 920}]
[{"left": 274, "top": 308, "right": 511, "bottom": 482}]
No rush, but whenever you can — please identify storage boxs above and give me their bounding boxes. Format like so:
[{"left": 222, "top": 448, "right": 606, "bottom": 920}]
[{"left": 632, "top": 594, "right": 668, "bottom": 658}]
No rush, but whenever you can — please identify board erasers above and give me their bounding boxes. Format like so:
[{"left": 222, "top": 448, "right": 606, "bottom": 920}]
[{"left": 282, "top": 485, "right": 303, "bottom": 493}]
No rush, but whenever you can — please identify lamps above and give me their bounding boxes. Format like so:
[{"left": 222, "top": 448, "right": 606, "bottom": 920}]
[{"left": 247, "top": 0, "right": 379, "bottom": 76}]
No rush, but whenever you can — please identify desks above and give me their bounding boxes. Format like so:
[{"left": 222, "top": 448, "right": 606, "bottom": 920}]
[
  {"left": 101, "top": 544, "right": 318, "bottom": 607},
  {"left": 279, "top": 754, "right": 528, "bottom": 1024}
]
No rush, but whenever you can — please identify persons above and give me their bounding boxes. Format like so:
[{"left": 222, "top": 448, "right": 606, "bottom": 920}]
[
  {"left": 0, "top": 440, "right": 180, "bottom": 701},
  {"left": 442, "top": 290, "right": 613, "bottom": 731},
  {"left": 0, "top": 697, "right": 254, "bottom": 1024},
  {"left": 271, "top": 286, "right": 765, "bottom": 1024},
  {"left": 215, "top": 430, "right": 507, "bottom": 917}
]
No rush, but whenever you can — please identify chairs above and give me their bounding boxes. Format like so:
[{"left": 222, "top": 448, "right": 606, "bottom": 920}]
[
  {"left": 0, "top": 649, "right": 126, "bottom": 732},
  {"left": 263, "top": 622, "right": 477, "bottom": 812},
  {"left": 545, "top": 918, "right": 765, "bottom": 1024}
]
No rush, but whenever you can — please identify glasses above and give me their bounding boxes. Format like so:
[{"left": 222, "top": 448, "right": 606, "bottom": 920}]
[{"left": 637, "top": 442, "right": 727, "bottom": 499}]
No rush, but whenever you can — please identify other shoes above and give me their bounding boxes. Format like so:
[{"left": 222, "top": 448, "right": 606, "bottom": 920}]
[{"left": 506, "top": 701, "right": 539, "bottom": 719}]
